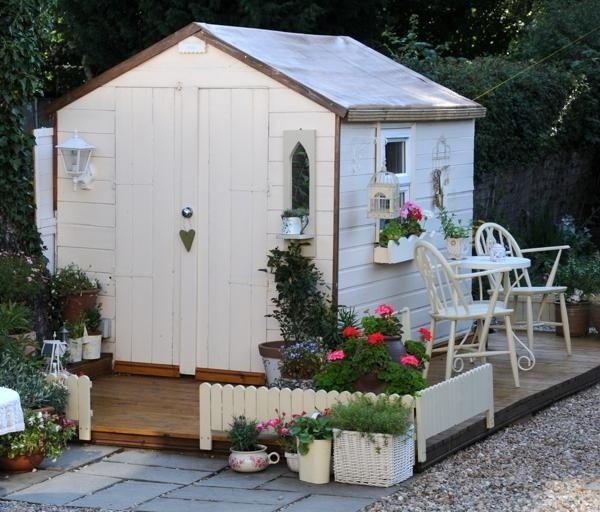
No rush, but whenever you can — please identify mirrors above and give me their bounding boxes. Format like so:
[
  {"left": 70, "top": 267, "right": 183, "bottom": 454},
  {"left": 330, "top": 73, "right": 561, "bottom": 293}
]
[{"left": 291, "top": 142, "right": 310, "bottom": 215}]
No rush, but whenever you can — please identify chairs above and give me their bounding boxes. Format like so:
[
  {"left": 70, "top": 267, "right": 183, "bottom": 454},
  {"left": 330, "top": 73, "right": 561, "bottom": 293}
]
[
  {"left": 412, "top": 239, "right": 522, "bottom": 389},
  {"left": 470, "top": 222, "right": 574, "bottom": 362}
]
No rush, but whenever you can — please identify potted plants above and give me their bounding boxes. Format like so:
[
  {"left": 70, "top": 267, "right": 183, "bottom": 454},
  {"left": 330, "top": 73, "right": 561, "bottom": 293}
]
[
  {"left": 542, "top": 255, "right": 600, "bottom": 336},
  {"left": 282, "top": 209, "right": 303, "bottom": 235},
  {"left": 329, "top": 393, "right": 415, "bottom": 487},
  {"left": 0, "top": 248, "right": 107, "bottom": 472},
  {"left": 224, "top": 413, "right": 279, "bottom": 473},
  {"left": 289, "top": 417, "right": 332, "bottom": 484},
  {"left": 258, "top": 243, "right": 339, "bottom": 389},
  {"left": 436, "top": 209, "right": 478, "bottom": 259}
]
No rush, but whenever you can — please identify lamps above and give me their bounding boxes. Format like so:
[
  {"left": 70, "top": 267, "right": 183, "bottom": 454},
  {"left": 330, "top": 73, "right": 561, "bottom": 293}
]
[{"left": 54, "top": 130, "right": 99, "bottom": 191}]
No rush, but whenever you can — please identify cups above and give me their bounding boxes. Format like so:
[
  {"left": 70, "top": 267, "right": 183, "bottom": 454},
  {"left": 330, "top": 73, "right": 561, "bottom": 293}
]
[{"left": 281, "top": 214, "right": 310, "bottom": 235}]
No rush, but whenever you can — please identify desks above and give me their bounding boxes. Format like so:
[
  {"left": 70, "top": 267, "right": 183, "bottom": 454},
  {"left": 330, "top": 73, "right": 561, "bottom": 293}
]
[{"left": 450, "top": 255, "right": 537, "bottom": 373}]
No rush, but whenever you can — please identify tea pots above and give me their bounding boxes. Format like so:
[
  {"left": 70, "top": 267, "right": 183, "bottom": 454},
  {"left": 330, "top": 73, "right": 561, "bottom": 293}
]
[{"left": 489, "top": 244, "right": 507, "bottom": 262}]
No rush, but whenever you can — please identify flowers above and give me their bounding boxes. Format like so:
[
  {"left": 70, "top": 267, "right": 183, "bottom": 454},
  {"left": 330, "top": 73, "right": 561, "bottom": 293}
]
[
  {"left": 399, "top": 201, "right": 426, "bottom": 224},
  {"left": 323, "top": 304, "right": 431, "bottom": 392},
  {"left": 259, "top": 409, "right": 306, "bottom": 453}
]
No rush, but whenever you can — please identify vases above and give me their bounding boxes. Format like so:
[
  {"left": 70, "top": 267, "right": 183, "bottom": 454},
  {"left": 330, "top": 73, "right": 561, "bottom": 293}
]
[{"left": 284, "top": 451, "right": 300, "bottom": 472}]
